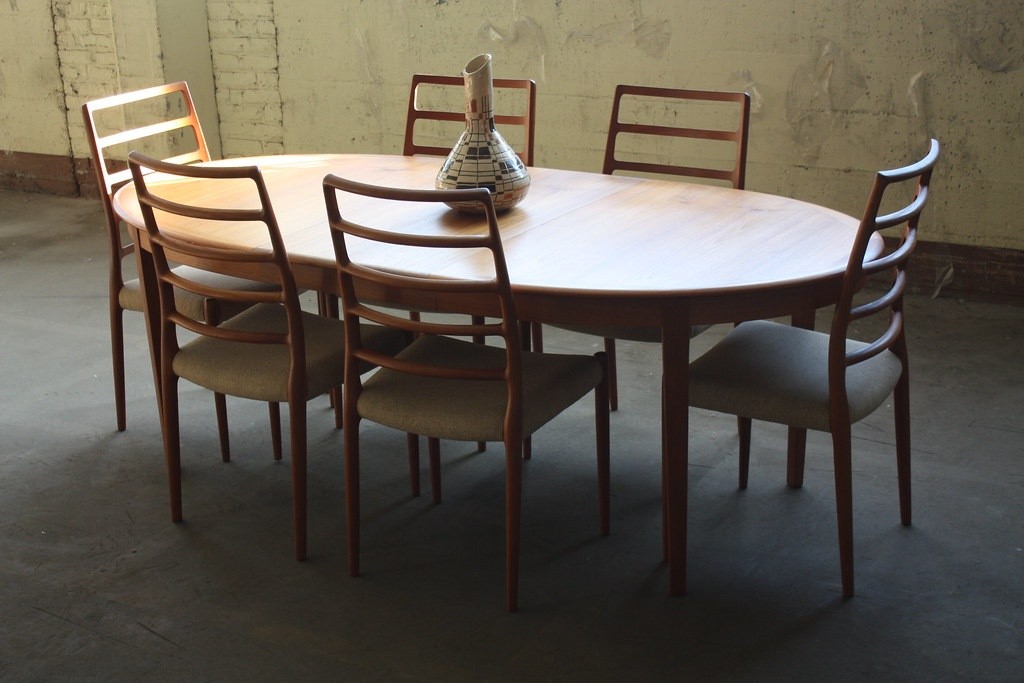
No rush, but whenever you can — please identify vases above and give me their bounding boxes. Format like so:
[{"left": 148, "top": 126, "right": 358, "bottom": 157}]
[{"left": 434, "top": 54, "right": 532, "bottom": 217}]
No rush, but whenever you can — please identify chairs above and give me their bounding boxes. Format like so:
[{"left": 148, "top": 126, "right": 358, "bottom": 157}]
[
  {"left": 602, "top": 82, "right": 751, "bottom": 410},
  {"left": 82, "top": 81, "right": 338, "bottom": 463},
  {"left": 665, "top": 138, "right": 941, "bottom": 604},
  {"left": 128, "top": 150, "right": 421, "bottom": 563},
  {"left": 323, "top": 174, "right": 613, "bottom": 614},
  {"left": 402, "top": 73, "right": 536, "bottom": 460}
]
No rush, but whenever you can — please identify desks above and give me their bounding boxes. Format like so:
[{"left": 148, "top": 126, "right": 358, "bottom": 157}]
[{"left": 112, "top": 154, "right": 883, "bottom": 602}]
